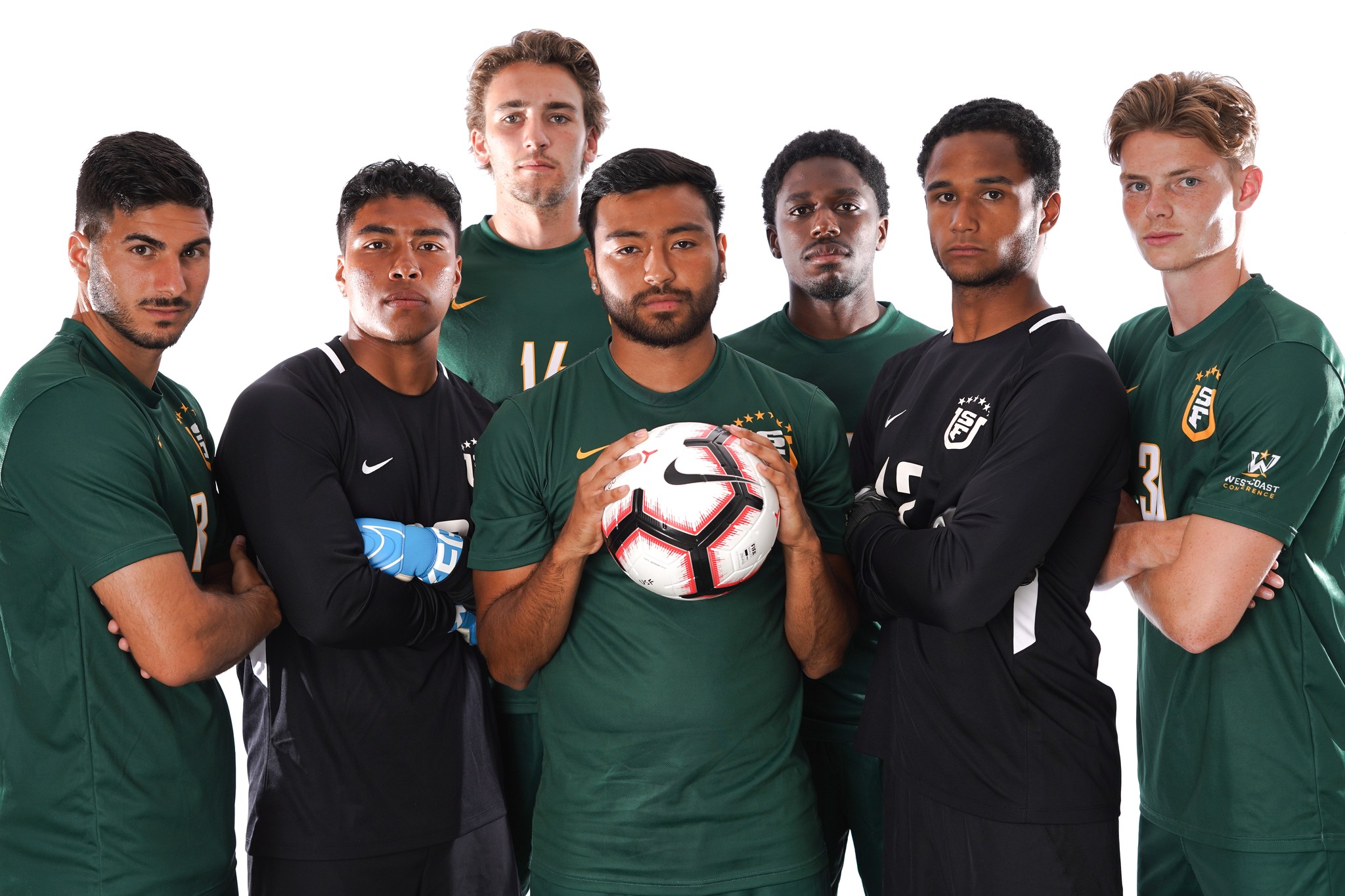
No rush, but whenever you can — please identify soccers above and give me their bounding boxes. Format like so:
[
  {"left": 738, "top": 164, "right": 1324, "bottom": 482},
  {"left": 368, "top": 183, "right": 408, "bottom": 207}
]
[{"left": 601, "top": 423, "right": 780, "bottom": 600}]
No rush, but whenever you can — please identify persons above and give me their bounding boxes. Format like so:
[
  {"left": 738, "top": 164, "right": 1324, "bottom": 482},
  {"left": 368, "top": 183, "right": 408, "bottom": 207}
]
[
  {"left": 1092, "top": 70, "right": 1344, "bottom": 896},
  {"left": 215, "top": 156, "right": 520, "bottom": 896},
  {"left": 468, "top": 146, "right": 849, "bottom": 896},
  {"left": 434, "top": 25, "right": 613, "bottom": 404},
  {"left": 0, "top": 133, "right": 283, "bottom": 896},
  {"left": 719, "top": 128, "right": 943, "bottom": 896},
  {"left": 848, "top": 93, "right": 1131, "bottom": 896}
]
